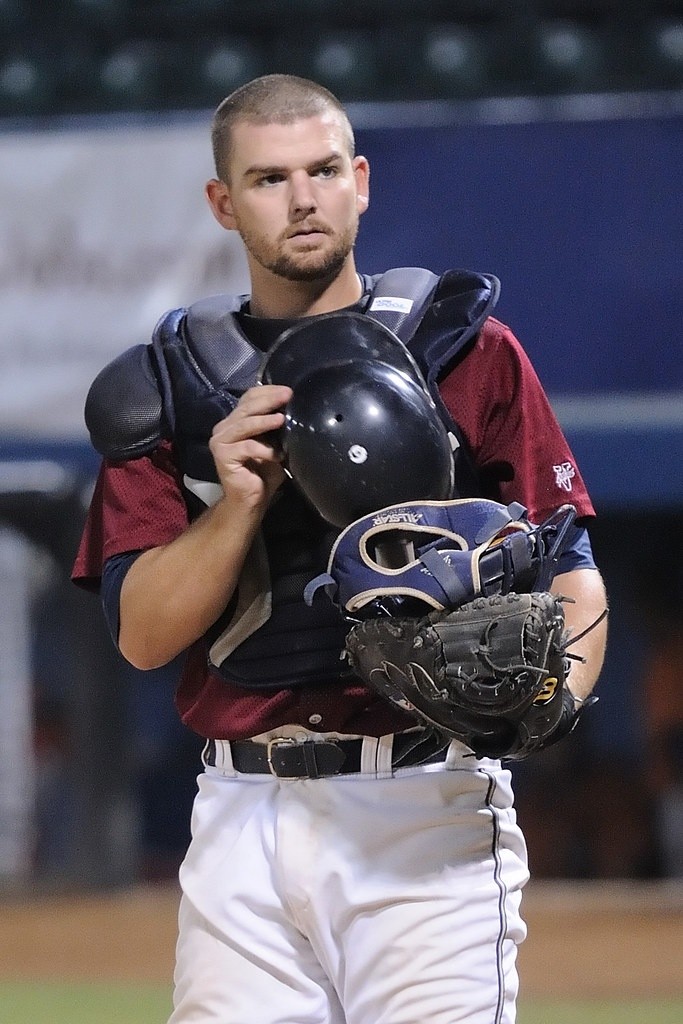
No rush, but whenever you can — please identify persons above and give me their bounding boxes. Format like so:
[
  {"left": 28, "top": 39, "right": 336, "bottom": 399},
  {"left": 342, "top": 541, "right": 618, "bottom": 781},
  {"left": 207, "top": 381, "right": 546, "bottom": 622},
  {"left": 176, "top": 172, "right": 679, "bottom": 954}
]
[{"left": 72, "top": 75, "right": 608, "bottom": 1024}]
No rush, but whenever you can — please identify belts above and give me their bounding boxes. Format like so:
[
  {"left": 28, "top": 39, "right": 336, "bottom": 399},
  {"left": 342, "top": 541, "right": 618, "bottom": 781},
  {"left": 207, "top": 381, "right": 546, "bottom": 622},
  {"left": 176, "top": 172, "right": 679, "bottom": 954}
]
[{"left": 203, "top": 728, "right": 449, "bottom": 780}]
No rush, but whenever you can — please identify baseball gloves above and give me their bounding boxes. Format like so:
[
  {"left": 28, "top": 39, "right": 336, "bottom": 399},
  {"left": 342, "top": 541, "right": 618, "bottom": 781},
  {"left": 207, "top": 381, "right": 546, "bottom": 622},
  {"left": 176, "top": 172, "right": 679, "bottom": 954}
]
[{"left": 344, "top": 592, "right": 584, "bottom": 766}]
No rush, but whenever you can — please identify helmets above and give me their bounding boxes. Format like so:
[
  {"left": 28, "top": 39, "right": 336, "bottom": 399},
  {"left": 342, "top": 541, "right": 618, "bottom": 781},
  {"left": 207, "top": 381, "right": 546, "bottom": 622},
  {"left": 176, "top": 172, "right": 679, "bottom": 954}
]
[{"left": 259, "top": 314, "right": 458, "bottom": 529}]
